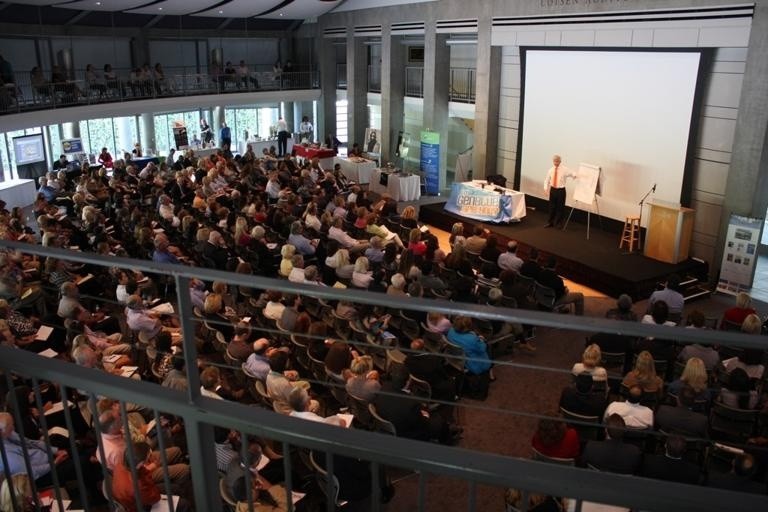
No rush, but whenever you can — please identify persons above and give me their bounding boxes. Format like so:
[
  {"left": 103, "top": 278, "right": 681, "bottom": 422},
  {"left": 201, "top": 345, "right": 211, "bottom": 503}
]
[
  {"left": 541, "top": 155, "right": 578, "bottom": 229},
  {"left": 0, "top": 112, "right": 567, "bottom": 512},
  {"left": 0, "top": 54, "right": 294, "bottom": 114},
  {"left": 508, "top": 271, "right": 767, "bottom": 511}
]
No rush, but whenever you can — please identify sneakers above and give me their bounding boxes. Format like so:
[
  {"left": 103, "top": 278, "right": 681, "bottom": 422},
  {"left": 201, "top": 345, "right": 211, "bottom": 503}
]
[
  {"left": 379, "top": 486, "right": 396, "bottom": 505},
  {"left": 489, "top": 374, "right": 496, "bottom": 382}
]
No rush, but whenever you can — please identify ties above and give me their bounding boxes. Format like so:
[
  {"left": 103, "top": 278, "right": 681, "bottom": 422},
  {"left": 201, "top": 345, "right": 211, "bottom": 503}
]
[{"left": 553, "top": 168, "right": 557, "bottom": 188}]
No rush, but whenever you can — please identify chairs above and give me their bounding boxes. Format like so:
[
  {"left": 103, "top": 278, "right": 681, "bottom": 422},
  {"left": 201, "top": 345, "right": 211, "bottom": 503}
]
[
  {"left": 4, "top": 70, "right": 278, "bottom": 109},
  {"left": 1, "top": 152, "right": 767, "bottom": 511}
]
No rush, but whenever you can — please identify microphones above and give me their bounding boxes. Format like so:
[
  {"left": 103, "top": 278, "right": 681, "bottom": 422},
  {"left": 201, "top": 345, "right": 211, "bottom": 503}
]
[{"left": 652, "top": 183, "right": 657, "bottom": 193}]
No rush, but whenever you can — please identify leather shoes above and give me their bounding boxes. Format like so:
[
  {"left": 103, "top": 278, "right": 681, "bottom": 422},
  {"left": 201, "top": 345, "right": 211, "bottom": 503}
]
[
  {"left": 558, "top": 307, "right": 569, "bottom": 314},
  {"left": 542, "top": 223, "right": 563, "bottom": 229},
  {"left": 520, "top": 342, "right": 537, "bottom": 353}
]
